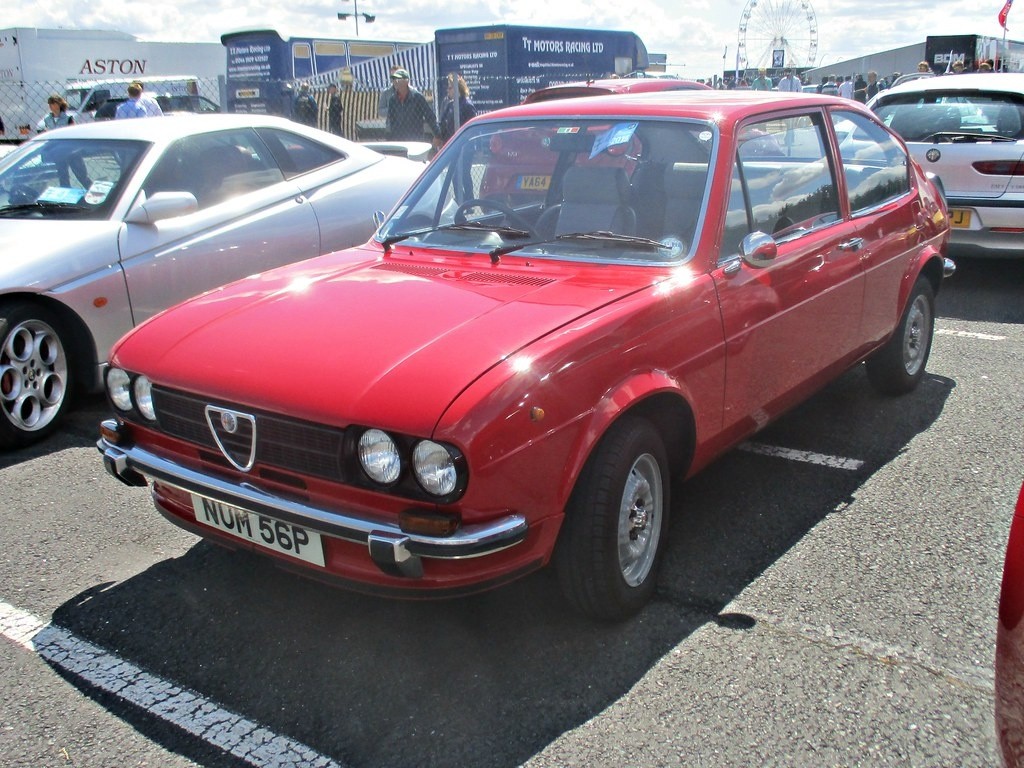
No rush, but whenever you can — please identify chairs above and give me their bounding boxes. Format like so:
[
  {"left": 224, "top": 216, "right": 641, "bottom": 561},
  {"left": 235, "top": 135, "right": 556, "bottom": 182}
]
[
  {"left": 660, "top": 161, "right": 708, "bottom": 238},
  {"left": 534, "top": 164, "right": 640, "bottom": 239}
]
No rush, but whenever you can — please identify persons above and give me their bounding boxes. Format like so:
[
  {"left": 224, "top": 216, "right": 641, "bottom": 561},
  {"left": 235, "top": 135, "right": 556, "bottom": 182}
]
[
  {"left": 328, "top": 83, "right": 342, "bottom": 137},
  {"left": 378, "top": 66, "right": 417, "bottom": 142},
  {"left": 0, "top": 116, "right": 5, "bottom": 136},
  {"left": 295, "top": 83, "right": 318, "bottom": 129},
  {"left": 114, "top": 83, "right": 148, "bottom": 120},
  {"left": 769, "top": 74, "right": 813, "bottom": 88},
  {"left": 778, "top": 69, "right": 802, "bottom": 144},
  {"left": 439, "top": 72, "right": 477, "bottom": 214},
  {"left": 132, "top": 80, "right": 164, "bottom": 117},
  {"left": 917, "top": 57, "right": 1008, "bottom": 105},
  {"left": 814, "top": 72, "right": 901, "bottom": 105},
  {"left": 44, "top": 95, "right": 93, "bottom": 190},
  {"left": 752, "top": 67, "right": 772, "bottom": 133},
  {"left": 697, "top": 76, "right": 753, "bottom": 90},
  {"left": 389, "top": 69, "right": 442, "bottom": 148}
]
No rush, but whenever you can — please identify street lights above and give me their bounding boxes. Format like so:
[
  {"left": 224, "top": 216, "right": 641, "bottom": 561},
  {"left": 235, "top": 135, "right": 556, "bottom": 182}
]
[{"left": 337, "top": 11, "right": 376, "bottom": 40}]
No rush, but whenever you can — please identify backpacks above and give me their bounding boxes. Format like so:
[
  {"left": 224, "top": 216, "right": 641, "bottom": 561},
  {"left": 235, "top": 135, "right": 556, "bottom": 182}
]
[{"left": 295, "top": 94, "right": 313, "bottom": 116}]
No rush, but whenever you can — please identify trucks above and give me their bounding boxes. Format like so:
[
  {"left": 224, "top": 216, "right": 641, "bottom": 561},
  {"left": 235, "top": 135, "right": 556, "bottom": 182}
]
[
  {"left": 436, "top": 22, "right": 651, "bottom": 148},
  {"left": 221, "top": 27, "right": 435, "bottom": 117}
]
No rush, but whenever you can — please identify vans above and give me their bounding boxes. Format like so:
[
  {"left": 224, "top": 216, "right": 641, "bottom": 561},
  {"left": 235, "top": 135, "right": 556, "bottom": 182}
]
[{"left": 35, "top": 76, "right": 202, "bottom": 134}]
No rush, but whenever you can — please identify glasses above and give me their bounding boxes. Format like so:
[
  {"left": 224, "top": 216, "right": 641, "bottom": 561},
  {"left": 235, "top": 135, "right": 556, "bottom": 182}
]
[
  {"left": 918, "top": 67, "right": 925, "bottom": 70},
  {"left": 48, "top": 100, "right": 59, "bottom": 104},
  {"left": 758, "top": 74, "right": 765, "bottom": 76}
]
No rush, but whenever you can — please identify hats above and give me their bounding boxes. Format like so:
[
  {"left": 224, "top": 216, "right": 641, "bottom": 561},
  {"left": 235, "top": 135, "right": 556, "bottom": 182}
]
[{"left": 389, "top": 68, "right": 410, "bottom": 79}]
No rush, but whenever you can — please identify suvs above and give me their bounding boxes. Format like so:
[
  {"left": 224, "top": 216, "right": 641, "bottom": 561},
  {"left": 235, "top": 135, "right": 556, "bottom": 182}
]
[
  {"left": 824, "top": 71, "right": 1024, "bottom": 282},
  {"left": 94, "top": 96, "right": 220, "bottom": 123}
]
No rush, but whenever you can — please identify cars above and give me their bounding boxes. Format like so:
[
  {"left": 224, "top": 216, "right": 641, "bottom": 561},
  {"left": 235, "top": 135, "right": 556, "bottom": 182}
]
[
  {"left": 0, "top": 113, "right": 459, "bottom": 447},
  {"left": 90, "top": 83, "right": 956, "bottom": 634},
  {"left": 478, "top": 78, "right": 770, "bottom": 228}
]
